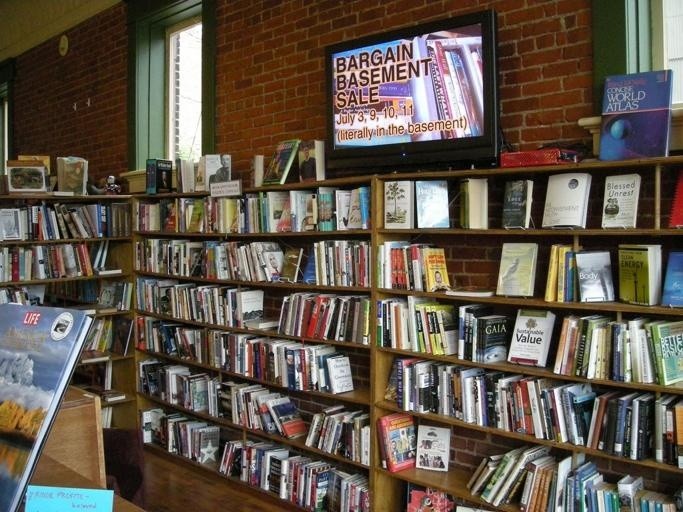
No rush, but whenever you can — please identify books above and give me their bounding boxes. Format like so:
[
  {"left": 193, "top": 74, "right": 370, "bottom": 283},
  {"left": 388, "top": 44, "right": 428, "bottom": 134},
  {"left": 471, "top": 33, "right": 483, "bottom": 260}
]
[
  {"left": 598, "top": 69, "right": 673, "bottom": 159},
  {"left": 426, "top": 41, "right": 485, "bottom": 139}
]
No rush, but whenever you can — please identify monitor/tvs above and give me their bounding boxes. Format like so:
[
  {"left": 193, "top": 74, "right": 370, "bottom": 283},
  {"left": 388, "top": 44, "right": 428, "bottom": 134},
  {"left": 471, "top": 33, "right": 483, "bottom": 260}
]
[{"left": 324, "top": 8, "right": 501, "bottom": 180}]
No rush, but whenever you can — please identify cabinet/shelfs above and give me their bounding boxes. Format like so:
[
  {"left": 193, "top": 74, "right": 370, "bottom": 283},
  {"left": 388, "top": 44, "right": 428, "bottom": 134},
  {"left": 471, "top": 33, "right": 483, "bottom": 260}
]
[{"left": 1, "top": 156, "right": 683, "bottom": 512}]
